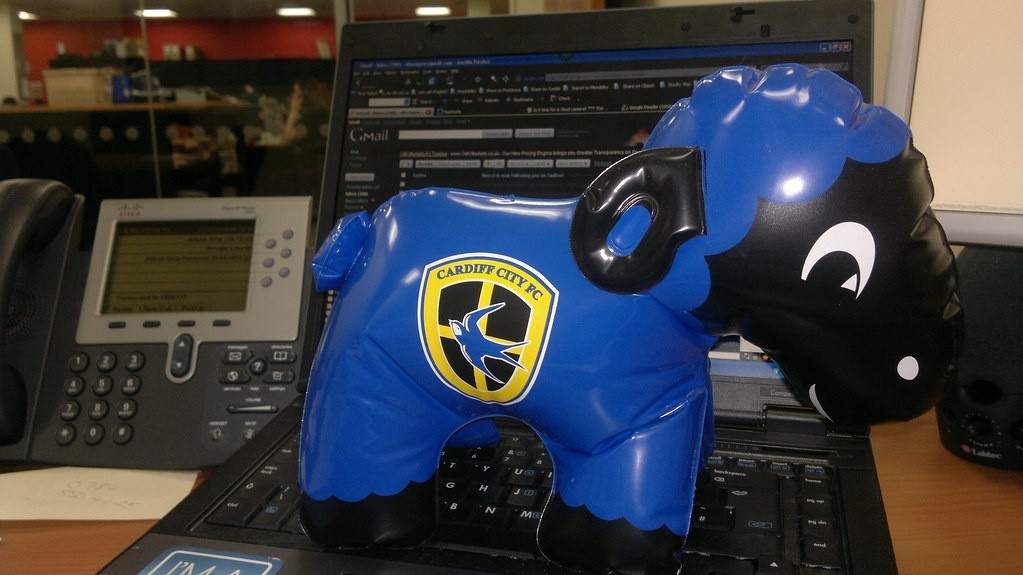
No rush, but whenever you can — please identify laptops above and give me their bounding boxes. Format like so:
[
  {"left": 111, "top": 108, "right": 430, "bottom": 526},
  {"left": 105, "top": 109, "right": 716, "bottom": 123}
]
[{"left": 98, "top": 0, "right": 899, "bottom": 575}]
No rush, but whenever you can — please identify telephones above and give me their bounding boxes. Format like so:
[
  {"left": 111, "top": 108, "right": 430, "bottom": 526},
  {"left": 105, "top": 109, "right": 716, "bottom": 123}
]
[{"left": 0, "top": 180, "right": 321, "bottom": 476}]
